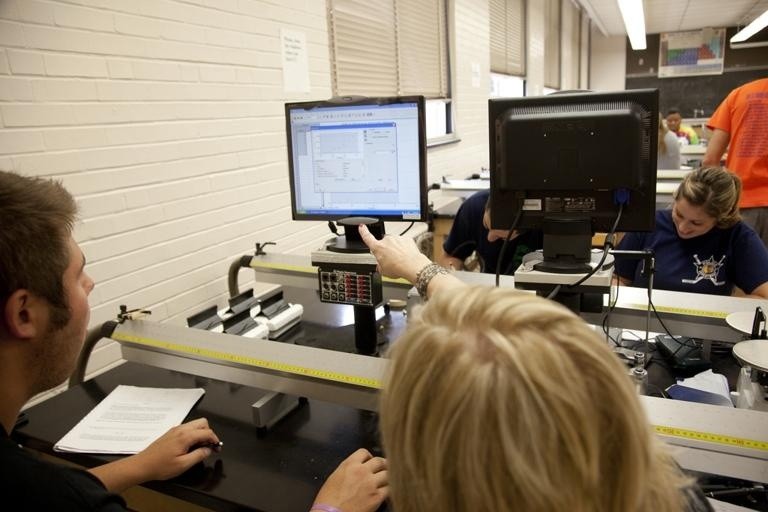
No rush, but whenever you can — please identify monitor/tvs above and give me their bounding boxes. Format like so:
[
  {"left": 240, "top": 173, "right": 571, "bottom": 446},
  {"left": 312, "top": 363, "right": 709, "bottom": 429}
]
[
  {"left": 488, "top": 88, "right": 658, "bottom": 273},
  {"left": 285, "top": 95, "right": 429, "bottom": 254}
]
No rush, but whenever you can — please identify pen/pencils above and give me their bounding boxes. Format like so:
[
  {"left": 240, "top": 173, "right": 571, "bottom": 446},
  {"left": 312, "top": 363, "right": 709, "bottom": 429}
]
[
  {"left": 449, "top": 262, "right": 457, "bottom": 271},
  {"left": 196, "top": 442, "right": 224, "bottom": 448}
]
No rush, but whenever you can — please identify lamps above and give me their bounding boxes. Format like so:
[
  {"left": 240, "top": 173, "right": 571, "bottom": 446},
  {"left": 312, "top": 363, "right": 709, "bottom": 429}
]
[{"left": 729, "top": 1, "right": 768, "bottom": 43}]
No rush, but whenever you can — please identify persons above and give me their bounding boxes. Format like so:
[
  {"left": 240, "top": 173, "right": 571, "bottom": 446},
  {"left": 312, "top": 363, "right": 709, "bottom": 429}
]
[
  {"left": 1, "top": 171, "right": 392, "bottom": 512},
  {"left": 356, "top": 223, "right": 714, "bottom": 510},
  {"left": 663, "top": 105, "right": 700, "bottom": 146},
  {"left": 656, "top": 112, "right": 681, "bottom": 170},
  {"left": 436, "top": 187, "right": 545, "bottom": 279},
  {"left": 702, "top": 78, "right": 767, "bottom": 250},
  {"left": 608, "top": 167, "right": 767, "bottom": 300}
]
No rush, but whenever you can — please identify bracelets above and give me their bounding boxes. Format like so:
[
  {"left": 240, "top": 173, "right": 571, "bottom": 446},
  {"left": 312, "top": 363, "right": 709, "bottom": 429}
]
[
  {"left": 416, "top": 262, "right": 451, "bottom": 305},
  {"left": 308, "top": 502, "right": 342, "bottom": 512}
]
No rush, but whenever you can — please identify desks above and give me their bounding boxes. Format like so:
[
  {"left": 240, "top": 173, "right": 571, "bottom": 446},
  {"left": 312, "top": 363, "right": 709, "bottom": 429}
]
[{"left": 10, "top": 285, "right": 410, "bottom": 512}]
